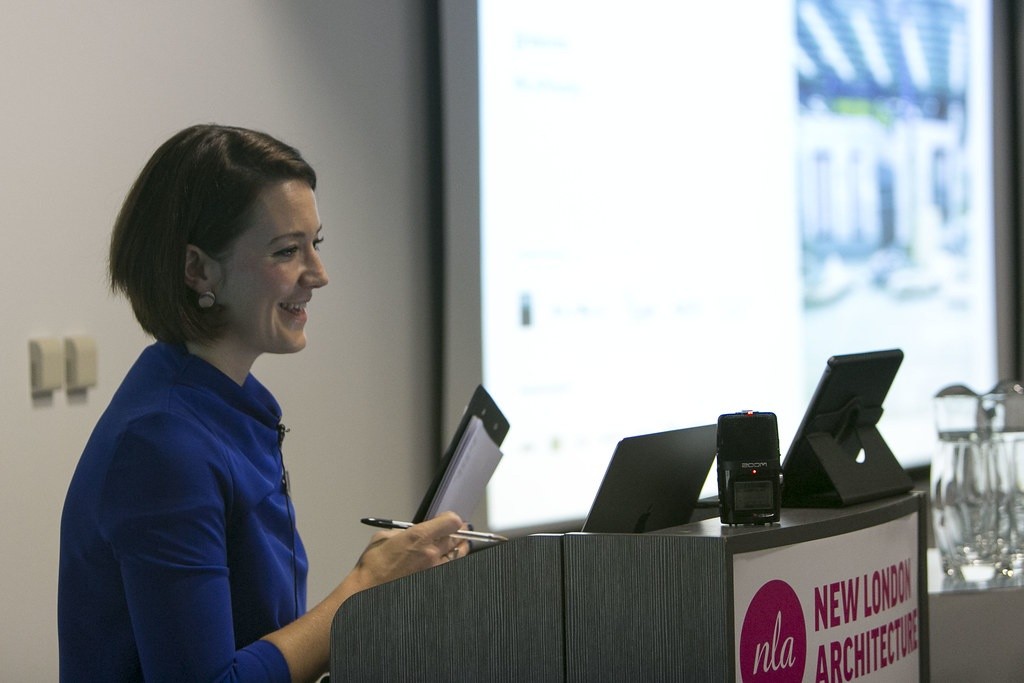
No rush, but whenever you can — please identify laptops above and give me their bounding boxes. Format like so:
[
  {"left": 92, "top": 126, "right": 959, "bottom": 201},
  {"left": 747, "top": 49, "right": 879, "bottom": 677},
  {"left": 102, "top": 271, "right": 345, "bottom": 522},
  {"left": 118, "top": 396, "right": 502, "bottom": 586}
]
[{"left": 580, "top": 424, "right": 718, "bottom": 534}]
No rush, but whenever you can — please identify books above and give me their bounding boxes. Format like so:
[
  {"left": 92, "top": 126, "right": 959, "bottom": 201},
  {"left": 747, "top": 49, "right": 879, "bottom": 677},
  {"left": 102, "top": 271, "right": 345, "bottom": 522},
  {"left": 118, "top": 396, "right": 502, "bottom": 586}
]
[{"left": 411, "top": 383, "right": 511, "bottom": 524}]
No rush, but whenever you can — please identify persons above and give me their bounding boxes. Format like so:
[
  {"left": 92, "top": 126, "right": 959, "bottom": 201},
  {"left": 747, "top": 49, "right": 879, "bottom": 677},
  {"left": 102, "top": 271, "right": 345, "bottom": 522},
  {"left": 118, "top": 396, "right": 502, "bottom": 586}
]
[{"left": 57, "top": 124, "right": 473, "bottom": 683}]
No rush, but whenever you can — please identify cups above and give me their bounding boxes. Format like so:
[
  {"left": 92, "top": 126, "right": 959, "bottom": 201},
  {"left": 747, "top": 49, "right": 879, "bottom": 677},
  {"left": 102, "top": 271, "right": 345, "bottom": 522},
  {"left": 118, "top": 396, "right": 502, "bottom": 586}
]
[{"left": 929, "top": 381, "right": 1024, "bottom": 583}]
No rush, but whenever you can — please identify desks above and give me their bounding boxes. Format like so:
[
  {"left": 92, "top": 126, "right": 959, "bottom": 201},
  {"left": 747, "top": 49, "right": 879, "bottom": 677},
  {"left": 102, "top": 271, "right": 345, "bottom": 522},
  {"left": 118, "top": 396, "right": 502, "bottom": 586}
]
[{"left": 924, "top": 545, "right": 1023, "bottom": 682}]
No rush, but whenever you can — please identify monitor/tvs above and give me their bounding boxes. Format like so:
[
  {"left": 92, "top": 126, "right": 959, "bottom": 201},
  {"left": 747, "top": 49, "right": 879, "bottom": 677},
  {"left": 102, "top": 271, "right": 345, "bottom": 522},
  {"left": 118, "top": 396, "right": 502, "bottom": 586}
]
[{"left": 779, "top": 349, "right": 904, "bottom": 507}]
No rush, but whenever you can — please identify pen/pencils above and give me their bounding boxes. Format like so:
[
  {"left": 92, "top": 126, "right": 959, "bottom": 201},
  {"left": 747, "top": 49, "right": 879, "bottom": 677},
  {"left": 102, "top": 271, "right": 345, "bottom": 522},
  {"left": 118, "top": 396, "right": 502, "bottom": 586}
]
[{"left": 360, "top": 517, "right": 509, "bottom": 545}]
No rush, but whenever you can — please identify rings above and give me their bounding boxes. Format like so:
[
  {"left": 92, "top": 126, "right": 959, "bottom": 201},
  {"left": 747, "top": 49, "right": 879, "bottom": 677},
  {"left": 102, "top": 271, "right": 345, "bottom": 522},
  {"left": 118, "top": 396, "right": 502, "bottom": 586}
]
[{"left": 445, "top": 548, "right": 458, "bottom": 561}]
